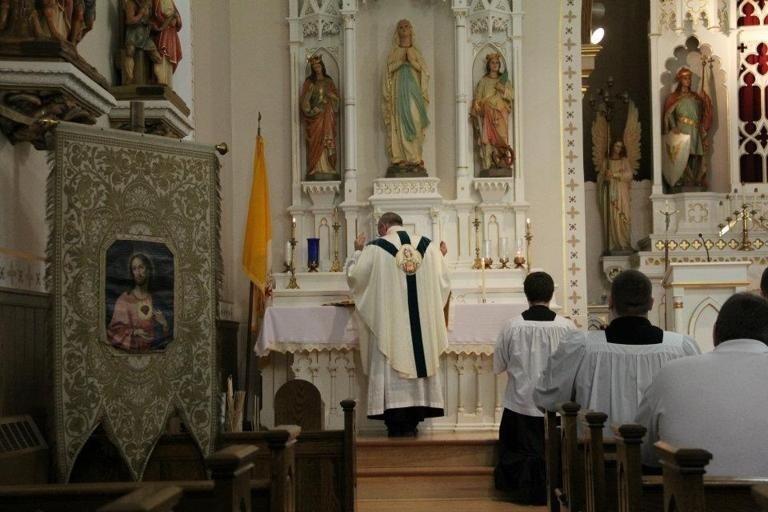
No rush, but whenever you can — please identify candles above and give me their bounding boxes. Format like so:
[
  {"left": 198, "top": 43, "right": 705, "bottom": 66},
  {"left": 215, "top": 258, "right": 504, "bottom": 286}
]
[
  {"left": 335, "top": 207, "right": 338, "bottom": 224},
  {"left": 292, "top": 217, "right": 296, "bottom": 238},
  {"left": 527, "top": 219, "right": 530, "bottom": 235},
  {"left": 475, "top": 206, "right": 478, "bottom": 219}
]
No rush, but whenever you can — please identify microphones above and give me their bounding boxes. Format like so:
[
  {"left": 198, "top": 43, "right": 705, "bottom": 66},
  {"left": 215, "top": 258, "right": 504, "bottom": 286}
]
[{"left": 698, "top": 231, "right": 712, "bottom": 263}]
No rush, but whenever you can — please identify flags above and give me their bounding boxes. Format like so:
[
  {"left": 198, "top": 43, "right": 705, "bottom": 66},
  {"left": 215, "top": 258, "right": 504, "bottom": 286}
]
[{"left": 237, "top": 133, "right": 269, "bottom": 343}]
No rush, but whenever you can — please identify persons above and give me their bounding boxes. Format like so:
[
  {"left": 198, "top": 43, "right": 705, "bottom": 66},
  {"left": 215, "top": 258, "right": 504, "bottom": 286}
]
[
  {"left": 595, "top": 140, "right": 634, "bottom": 248},
  {"left": 631, "top": 291, "right": 767, "bottom": 480},
  {"left": 347, "top": 211, "right": 453, "bottom": 435},
  {"left": 468, "top": 53, "right": 514, "bottom": 176},
  {"left": 107, "top": 252, "right": 172, "bottom": 349},
  {"left": 299, "top": 54, "right": 339, "bottom": 181},
  {"left": 662, "top": 66, "right": 713, "bottom": 189},
  {"left": 529, "top": 270, "right": 698, "bottom": 461},
  {"left": 490, "top": 271, "right": 584, "bottom": 505},
  {"left": 380, "top": 18, "right": 429, "bottom": 177},
  {"left": 114, "top": 1, "right": 183, "bottom": 84}
]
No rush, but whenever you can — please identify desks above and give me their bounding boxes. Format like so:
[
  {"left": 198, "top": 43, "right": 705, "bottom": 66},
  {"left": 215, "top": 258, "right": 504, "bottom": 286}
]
[{"left": 253, "top": 301, "right": 563, "bottom": 435}]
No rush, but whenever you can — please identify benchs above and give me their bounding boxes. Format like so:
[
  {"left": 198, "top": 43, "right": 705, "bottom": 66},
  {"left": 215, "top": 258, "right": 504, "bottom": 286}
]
[
  {"left": 0, "top": 399, "right": 357, "bottom": 512},
  {"left": 544, "top": 401, "right": 768, "bottom": 512}
]
